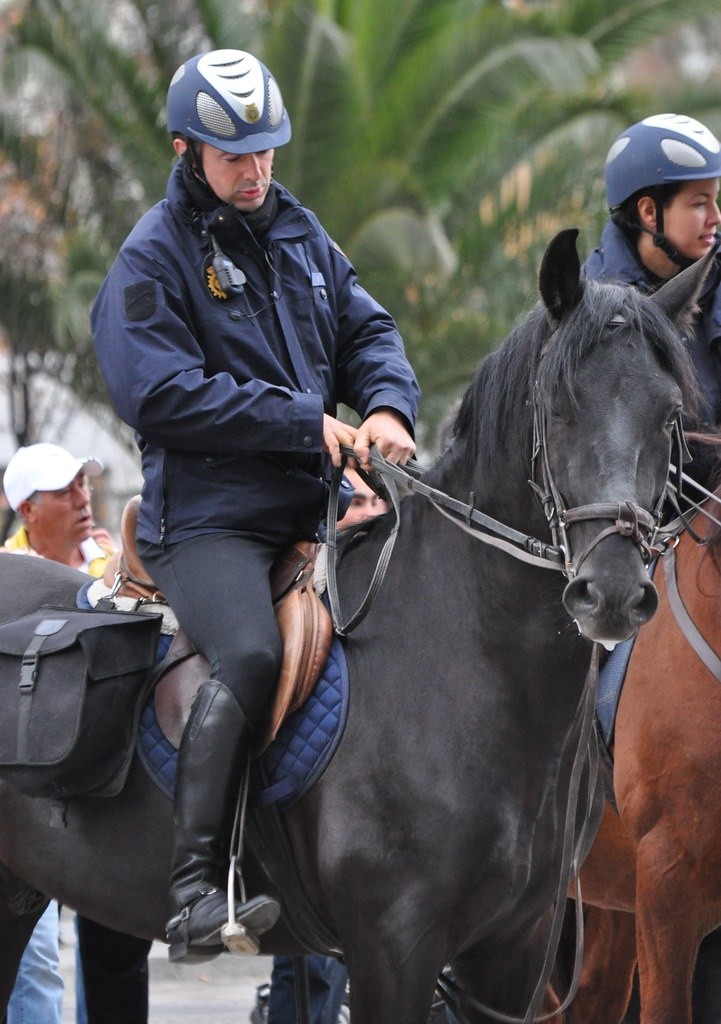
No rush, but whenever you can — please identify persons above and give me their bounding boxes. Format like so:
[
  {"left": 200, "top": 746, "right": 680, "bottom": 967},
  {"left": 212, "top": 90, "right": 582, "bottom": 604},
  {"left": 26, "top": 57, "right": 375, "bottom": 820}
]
[
  {"left": 91, "top": 50, "right": 422, "bottom": 967},
  {"left": 579, "top": 113, "right": 721, "bottom": 430},
  {"left": 1, "top": 444, "right": 151, "bottom": 1023}
]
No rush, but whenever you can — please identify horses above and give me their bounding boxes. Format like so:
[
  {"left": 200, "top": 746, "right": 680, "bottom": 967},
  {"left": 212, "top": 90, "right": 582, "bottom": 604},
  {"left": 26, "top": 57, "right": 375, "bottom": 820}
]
[{"left": 2, "top": 226, "right": 721, "bottom": 1023}]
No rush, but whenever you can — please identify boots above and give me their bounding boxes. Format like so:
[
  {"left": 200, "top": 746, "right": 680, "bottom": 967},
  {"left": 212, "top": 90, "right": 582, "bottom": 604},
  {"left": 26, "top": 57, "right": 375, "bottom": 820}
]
[{"left": 166, "top": 680, "right": 280, "bottom": 964}]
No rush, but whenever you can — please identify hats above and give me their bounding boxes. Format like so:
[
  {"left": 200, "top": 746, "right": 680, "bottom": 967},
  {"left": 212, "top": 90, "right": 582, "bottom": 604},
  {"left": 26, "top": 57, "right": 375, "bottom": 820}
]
[{"left": 3, "top": 443, "right": 103, "bottom": 511}]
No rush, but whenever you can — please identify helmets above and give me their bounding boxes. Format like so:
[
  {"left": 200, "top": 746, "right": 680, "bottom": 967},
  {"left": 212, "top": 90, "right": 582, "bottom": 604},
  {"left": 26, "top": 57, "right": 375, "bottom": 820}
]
[
  {"left": 605, "top": 113, "right": 721, "bottom": 213},
  {"left": 167, "top": 49, "right": 291, "bottom": 155}
]
